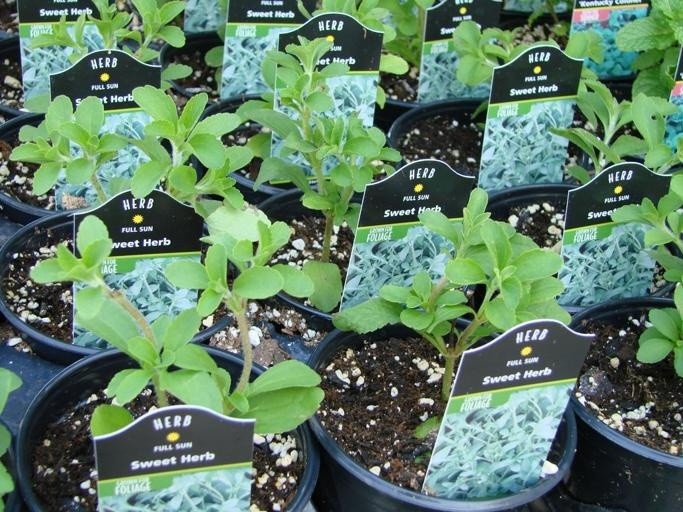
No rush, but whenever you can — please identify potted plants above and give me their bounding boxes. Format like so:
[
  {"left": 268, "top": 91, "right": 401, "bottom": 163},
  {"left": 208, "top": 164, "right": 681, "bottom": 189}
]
[{"left": 3, "top": 2, "right": 680, "bottom": 511}]
